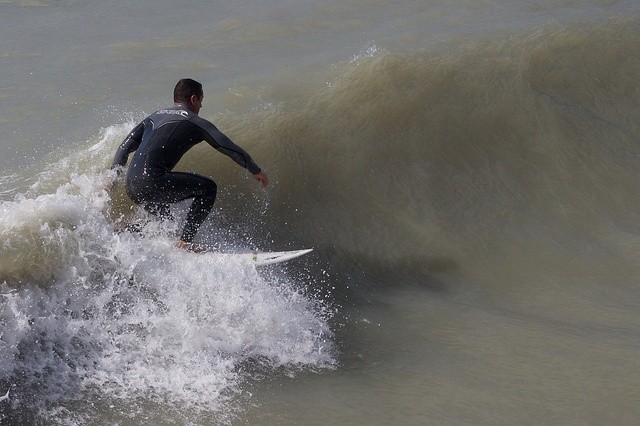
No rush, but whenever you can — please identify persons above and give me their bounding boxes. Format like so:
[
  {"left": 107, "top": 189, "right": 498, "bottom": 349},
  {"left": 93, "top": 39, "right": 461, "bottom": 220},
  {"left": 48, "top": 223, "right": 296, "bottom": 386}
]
[{"left": 110, "top": 78, "right": 269, "bottom": 253}]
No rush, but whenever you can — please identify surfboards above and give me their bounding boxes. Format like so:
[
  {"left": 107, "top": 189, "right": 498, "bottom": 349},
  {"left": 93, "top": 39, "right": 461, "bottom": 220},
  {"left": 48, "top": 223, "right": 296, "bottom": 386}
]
[{"left": 206, "top": 249, "right": 315, "bottom": 266}]
